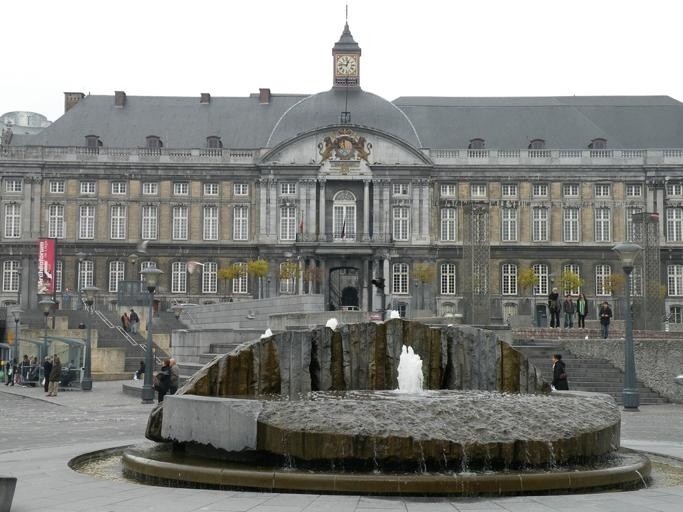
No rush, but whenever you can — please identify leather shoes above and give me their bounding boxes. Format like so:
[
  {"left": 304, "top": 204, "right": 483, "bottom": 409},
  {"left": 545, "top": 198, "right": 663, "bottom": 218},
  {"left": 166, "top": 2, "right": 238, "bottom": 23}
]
[{"left": 5, "top": 384, "right": 14, "bottom": 387}]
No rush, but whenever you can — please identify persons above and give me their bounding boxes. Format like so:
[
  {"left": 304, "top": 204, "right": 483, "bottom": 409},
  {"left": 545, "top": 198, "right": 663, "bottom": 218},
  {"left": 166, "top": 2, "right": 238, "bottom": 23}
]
[
  {"left": 550, "top": 352, "right": 570, "bottom": 390},
  {"left": 5, "top": 353, "right": 61, "bottom": 398},
  {"left": 548, "top": 287, "right": 613, "bottom": 339},
  {"left": 153, "top": 358, "right": 180, "bottom": 402},
  {"left": 135, "top": 360, "right": 147, "bottom": 379},
  {"left": 120, "top": 308, "right": 141, "bottom": 335},
  {"left": 63, "top": 288, "right": 72, "bottom": 309}
]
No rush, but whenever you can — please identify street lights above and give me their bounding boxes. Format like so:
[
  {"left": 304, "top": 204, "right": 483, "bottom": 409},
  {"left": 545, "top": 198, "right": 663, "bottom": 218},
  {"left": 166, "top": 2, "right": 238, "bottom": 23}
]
[
  {"left": 78, "top": 285, "right": 104, "bottom": 390},
  {"left": 610, "top": 243, "right": 647, "bottom": 412},
  {"left": 35, "top": 299, "right": 57, "bottom": 358},
  {"left": 10, "top": 309, "right": 25, "bottom": 362},
  {"left": 138, "top": 268, "right": 165, "bottom": 404}
]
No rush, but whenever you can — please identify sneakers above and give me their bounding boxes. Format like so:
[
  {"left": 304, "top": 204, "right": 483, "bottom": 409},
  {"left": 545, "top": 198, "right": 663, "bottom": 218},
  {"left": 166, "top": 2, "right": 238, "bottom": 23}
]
[
  {"left": 17, "top": 385, "right": 27, "bottom": 388},
  {"left": 549, "top": 326, "right": 588, "bottom": 330},
  {"left": 45, "top": 394, "right": 57, "bottom": 397}
]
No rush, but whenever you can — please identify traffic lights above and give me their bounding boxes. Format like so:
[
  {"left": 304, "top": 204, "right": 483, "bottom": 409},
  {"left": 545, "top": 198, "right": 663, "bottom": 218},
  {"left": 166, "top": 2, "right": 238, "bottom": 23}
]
[{"left": 371, "top": 255, "right": 386, "bottom": 322}]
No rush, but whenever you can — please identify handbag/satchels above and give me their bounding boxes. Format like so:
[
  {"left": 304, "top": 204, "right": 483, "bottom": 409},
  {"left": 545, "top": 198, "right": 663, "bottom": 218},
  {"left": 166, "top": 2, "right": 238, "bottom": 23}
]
[{"left": 153, "top": 377, "right": 160, "bottom": 387}]
